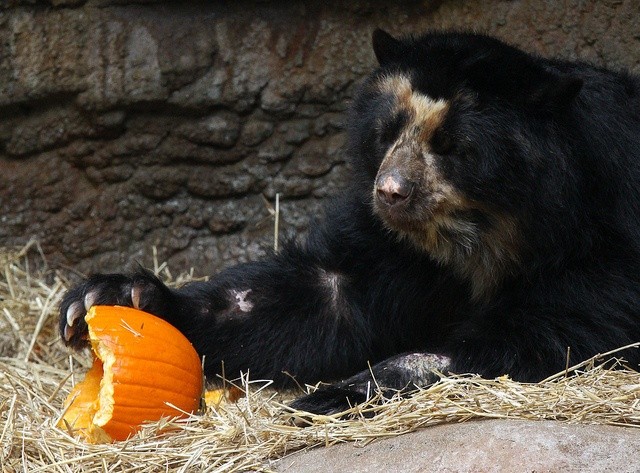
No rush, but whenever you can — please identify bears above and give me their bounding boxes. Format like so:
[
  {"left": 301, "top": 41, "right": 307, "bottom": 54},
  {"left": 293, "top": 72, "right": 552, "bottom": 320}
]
[{"left": 58, "top": 27, "right": 640, "bottom": 428}]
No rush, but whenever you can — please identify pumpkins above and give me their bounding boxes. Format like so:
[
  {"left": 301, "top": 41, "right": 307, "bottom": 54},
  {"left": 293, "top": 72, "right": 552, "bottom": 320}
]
[{"left": 57, "top": 304, "right": 204, "bottom": 443}]
